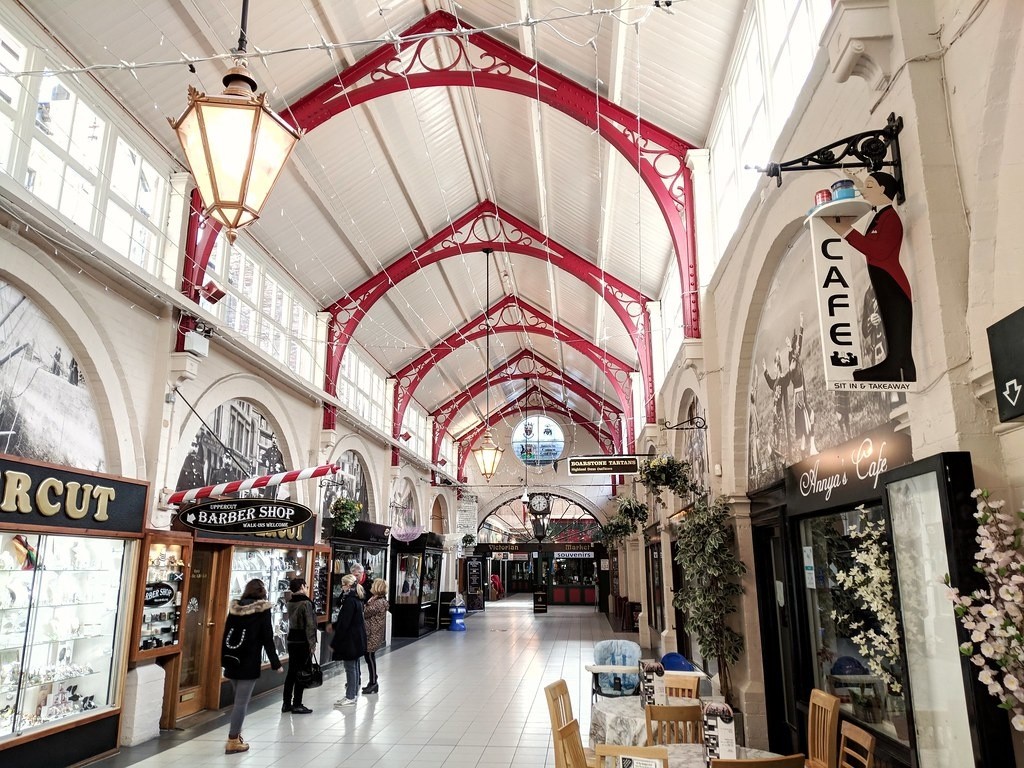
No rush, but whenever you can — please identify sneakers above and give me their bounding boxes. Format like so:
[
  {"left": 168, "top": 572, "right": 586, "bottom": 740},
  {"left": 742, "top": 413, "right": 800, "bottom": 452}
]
[
  {"left": 282, "top": 703, "right": 293, "bottom": 712},
  {"left": 291, "top": 705, "right": 312, "bottom": 713},
  {"left": 334, "top": 694, "right": 357, "bottom": 707}
]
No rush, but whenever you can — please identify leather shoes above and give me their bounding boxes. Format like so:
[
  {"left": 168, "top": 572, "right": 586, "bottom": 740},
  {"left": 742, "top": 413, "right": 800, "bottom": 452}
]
[{"left": 225, "top": 734, "right": 249, "bottom": 753}]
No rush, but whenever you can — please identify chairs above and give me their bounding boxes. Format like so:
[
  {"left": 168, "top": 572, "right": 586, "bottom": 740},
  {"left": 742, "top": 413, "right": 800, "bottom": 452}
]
[
  {"left": 594, "top": 639, "right": 642, "bottom": 699},
  {"left": 709, "top": 753, "right": 805, "bottom": 768},
  {"left": 655, "top": 675, "right": 698, "bottom": 700},
  {"left": 805, "top": 688, "right": 839, "bottom": 768},
  {"left": 837, "top": 720, "right": 876, "bottom": 768},
  {"left": 595, "top": 744, "right": 669, "bottom": 768},
  {"left": 545, "top": 678, "right": 595, "bottom": 768},
  {"left": 644, "top": 706, "right": 704, "bottom": 744}
]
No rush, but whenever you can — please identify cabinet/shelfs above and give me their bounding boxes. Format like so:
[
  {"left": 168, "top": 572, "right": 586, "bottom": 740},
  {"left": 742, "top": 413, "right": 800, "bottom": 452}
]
[
  {"left": 142, "top": 560, "right": 188, "bottom": 636},
  {"left": 0, "top": 567, "right": 118, "bottom": 695}
]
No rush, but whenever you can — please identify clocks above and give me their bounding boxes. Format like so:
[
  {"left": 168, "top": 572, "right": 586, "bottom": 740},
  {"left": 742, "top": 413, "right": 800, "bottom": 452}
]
[{"left": 531, "top": 494, "right": 550, "bottom": 513}]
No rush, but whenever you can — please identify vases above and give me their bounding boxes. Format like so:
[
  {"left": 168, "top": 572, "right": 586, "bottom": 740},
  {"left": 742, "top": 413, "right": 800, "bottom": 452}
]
[
  {"left": 609, "top": 525, "right": 623, "bottom": 535},
  {"left": 649, "top": 468, "right": 673, "bottom": 487},
  {"left": 463, "top": 544, "right": 477, "bottom": 556},
  {"left": 622, "top": 505, "right": 640, "bottom": 516}
]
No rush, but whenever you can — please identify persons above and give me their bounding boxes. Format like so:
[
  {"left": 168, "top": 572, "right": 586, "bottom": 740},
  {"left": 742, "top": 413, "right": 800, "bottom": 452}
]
[
  {"left": 344, "top": 563, "right": 374, "bottom": 687},
  {"left": 281, "top": 577, "right": 318, "bottom": 714},
  {"left": 748, "top": 310, "right": 853, "bottom": 470},
  {"left": 323, "top": 478, "right": 369, "bottom": 522},
  {"left": 173, "top": 432, "right": 287, "bottom": 507},
  {"left": 326, "top": 573, "right": 367, "bottom": 707},
  {"left": 222, "top": 578, "right": 284, "bottom": 754},
  {"left": 361, "top": 577, "right": 390, "bottom": 694}
]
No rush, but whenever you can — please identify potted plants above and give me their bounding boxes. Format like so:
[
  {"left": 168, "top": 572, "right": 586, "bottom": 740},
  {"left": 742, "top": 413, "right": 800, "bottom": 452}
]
[{"left": 671, "top": 494, "right": 748, "bottom": 746}]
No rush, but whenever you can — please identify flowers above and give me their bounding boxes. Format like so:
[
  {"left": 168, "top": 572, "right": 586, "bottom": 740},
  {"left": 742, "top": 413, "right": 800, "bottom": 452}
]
[
  {"left": 639, "top": 454, "right": 698, "bottom": 507},
  {"left": 463, "top": 534, "right": 475, "bottom": 545},
  {"left": 618, "top": 497, "right": 653, "bottom": 533},
  {"left": 605, "top": 519, "right": 635, "bottom": 540},
  {"left": 329, "top": 497, "right": 364, "bottom": 533}
]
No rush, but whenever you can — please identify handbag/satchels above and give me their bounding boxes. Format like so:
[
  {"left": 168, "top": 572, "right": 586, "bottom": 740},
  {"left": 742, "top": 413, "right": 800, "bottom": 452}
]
[{"left": 296, "top": 652, "right": 323, "bottom": 688}]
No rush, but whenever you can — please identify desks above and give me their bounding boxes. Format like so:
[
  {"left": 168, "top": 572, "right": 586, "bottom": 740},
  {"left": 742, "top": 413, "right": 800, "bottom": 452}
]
[
  {"left": 584, "top": 665, "right": 638, "bottom": 674},
  {"left": 589, "top": 693, "right": 705, "bottom": 752},
  {"left": 623, "top": 744, "right": 783, "bottom": 768}
]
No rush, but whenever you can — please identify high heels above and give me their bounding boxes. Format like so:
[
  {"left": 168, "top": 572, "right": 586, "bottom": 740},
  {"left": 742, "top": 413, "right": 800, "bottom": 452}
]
[{"left": 362, "top": 682, "right": 378, "bottom": 694}]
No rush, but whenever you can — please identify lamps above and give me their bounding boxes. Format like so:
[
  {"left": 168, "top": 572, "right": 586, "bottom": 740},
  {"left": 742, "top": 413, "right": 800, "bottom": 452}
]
[
  {"left": 183, "top": 323, "right": 215, "bottom": 357},
  {"left": 471, "top": 246, "right": 506, "bottom": 483},
  {"left": 400, "top": 431, "right": 411, "bottom": 442},
  {"left": 438, "top": 459, "right": 447, "bottom": 467},
  {"left": 165, "top": 2, "right": 302, "bottom": 244},
  {"left": 462, "top": 477, "right": 468, "bottom": 484},
  {"left": 193, "top": 281, "right": 226, "bottom": 305}
]
